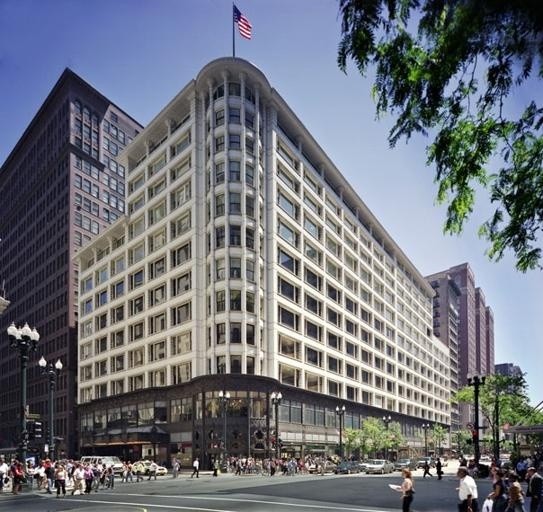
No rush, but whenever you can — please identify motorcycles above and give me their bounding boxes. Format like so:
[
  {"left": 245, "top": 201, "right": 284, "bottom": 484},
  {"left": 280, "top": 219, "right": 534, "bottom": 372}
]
[{"left": 457, "top": 457, "right": 479, "bottom": 479}]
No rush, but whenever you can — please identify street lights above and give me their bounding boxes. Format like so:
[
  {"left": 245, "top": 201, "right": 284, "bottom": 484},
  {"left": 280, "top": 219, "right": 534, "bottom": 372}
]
[
  {"left": 219, "top": 391, "right": 230, "bottom": 454},
  {"left": 6, "top": 321, "right": 40, "bottom": 482},
  {"left": 421, "top": 423, "right": 431, "bottom": 455},
  {"left": 467, "top": 371, "right": 486, "bottom": 463},
  {"left": 270, "top": 392, "right": 283, "bottom": 457},
  {"left": 382, "top": 415, "right": 391, "bottom": 459},
  {"left": 336, "top": 405, "right": 346, "bottom": 457},
  {"left": 38, "top": 357, "right": 62, "bottom": 461}
]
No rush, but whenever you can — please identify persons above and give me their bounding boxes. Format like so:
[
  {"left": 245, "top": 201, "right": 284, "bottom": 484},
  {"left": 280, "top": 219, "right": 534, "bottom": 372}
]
[
  {"left": 435, "top": 458, "right": 442, "bottom": 480},
  {"left": 170, "top": 454, "right": 360, "bottom": 481},
  {"left": 454, "top": 454, "right": 542, "bottom": 512},
  {"left": 0, "top": 451, "right": 158, "bottom": 498},
  {"left": 395, "top": 469, "right": 415, "bottom": 512},
  {"left": 421, "top": 461, "right": 433, "bottom": 478}
]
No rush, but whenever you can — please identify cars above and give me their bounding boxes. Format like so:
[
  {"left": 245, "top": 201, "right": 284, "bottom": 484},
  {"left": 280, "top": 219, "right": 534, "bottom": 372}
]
[
  {"left": 132, "top": 461, "right": 167, "bottom": 476},
  {"left": 308, "top": 461, "right": 337, "bottom": 474},
  {"left": 418, "top": 457, "right": 448, "bottom": 468},
  {"left": 337, "top": 458, "right": 416, "bottom": 475}
]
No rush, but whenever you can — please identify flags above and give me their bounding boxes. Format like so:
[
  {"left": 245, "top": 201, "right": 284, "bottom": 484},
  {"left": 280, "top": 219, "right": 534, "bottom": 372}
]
[{"left": 233, "top": 4, "right": 251, "bottom": 39}]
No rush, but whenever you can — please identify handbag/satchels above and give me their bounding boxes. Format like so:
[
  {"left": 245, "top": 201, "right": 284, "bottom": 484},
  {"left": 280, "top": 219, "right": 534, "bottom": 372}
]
[
  {"left": 441, "top": 471, "right": 443, "bottom": 474},
  {"left": 406, "top": 489, "right": 414, "bottom": 494}
]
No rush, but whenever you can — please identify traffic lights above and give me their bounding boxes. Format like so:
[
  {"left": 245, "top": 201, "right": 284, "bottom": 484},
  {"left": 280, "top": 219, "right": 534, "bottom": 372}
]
[{"left": 472, "top": 431, "right": 478, "bottom": 444}]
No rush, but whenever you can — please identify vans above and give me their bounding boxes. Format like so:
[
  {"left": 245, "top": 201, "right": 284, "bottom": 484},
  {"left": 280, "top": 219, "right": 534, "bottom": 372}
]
[{"left": 81, "top": 456, "right": 124, "bottom": 477}]
